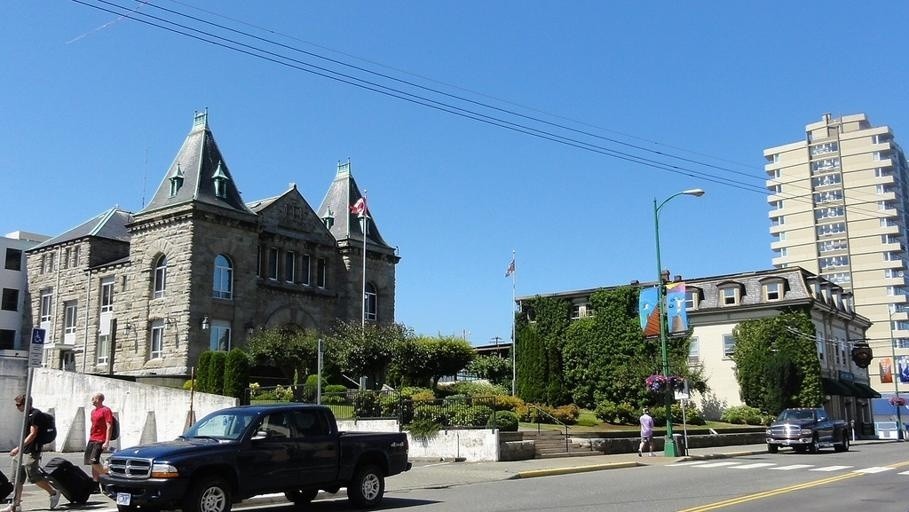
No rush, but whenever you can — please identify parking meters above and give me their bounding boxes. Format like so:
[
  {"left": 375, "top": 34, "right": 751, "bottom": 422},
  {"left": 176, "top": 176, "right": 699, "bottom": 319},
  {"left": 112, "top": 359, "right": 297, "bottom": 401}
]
[{"left": 851, "top": 419, "right": 856, "bottom": 441}]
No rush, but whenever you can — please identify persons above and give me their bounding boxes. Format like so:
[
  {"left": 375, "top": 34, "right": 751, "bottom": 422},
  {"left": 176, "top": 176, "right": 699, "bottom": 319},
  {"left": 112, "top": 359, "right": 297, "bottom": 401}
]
[
  {"left": 638, "top": 408, "right": 657, "bottom": 457},
  {"left": 0, "top": 393, "right": 61, "bottom": 512},
  {"left": 83, "top": 393, "right": 120, "bottom": 493}
]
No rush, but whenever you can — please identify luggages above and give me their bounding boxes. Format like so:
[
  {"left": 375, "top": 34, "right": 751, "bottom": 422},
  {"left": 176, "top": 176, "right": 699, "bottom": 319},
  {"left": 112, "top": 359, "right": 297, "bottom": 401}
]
[
  {"left": 38, "top": 457, "right": 94, "bottom": 505},
  {"left": 0, "top": 471, "right": 13, "bottom": 502}
]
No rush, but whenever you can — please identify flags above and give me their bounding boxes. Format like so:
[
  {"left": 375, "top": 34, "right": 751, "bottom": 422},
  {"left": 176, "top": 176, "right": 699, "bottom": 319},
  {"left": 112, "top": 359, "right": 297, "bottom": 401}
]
[
  {"left": 504, "top": 258, "right": 515, "bottom": 278},
  {"left": 349, "top": 195, "right": 365, "bottom": 215}
]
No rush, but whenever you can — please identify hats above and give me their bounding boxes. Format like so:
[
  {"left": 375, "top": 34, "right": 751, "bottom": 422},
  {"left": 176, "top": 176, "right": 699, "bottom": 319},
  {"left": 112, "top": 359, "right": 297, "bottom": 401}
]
[{"left": 643, "top": 408, "right": 649, "bottom": 414}]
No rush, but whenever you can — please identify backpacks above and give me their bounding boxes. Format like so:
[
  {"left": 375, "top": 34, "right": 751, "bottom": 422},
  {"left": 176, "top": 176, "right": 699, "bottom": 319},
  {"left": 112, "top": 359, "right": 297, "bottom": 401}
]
[{"left": 29, "top": 409, "right": 56, "bottom": 444}]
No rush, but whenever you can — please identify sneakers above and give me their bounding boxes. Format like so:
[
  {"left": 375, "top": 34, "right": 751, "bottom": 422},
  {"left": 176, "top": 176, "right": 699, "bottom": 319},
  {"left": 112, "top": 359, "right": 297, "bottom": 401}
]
[
  {"left": 91, "top": 487, "right": 100, "bottom": 493},
  {"left": 49, "top": 490, "right": 61, "bottom": 508},
  {"left": 1, "top": 504, "right": 21, "bottom": 512},
  {"left": 649, "top": 453, "right": 656, "bottom": 456},
  {"left": 638, "top": 449, "right": 643, "bottom": 457}
]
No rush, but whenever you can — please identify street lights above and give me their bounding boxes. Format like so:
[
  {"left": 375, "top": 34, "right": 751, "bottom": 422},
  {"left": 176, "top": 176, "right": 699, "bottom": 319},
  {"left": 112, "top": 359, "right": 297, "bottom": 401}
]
[
  {"left": 888, "top": 305, "right": 909, "bottom": 443},
  {"left": 653, "top": 187, "right": 705, "bottom": 457}
]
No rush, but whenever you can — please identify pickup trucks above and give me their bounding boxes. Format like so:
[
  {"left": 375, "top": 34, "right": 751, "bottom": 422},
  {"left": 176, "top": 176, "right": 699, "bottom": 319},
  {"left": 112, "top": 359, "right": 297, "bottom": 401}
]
[
  {"left": 766, "top": 406, "right": 849, "bottom": 453},
  {"left": 98, "top": 403, "right": 412, "bottom": 512}
]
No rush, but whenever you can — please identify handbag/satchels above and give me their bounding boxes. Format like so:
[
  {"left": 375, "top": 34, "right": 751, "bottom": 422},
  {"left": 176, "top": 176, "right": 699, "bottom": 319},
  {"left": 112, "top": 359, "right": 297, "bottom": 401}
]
[{"left": 110, "top": 415, "right": 119, "bottom": 440}]
